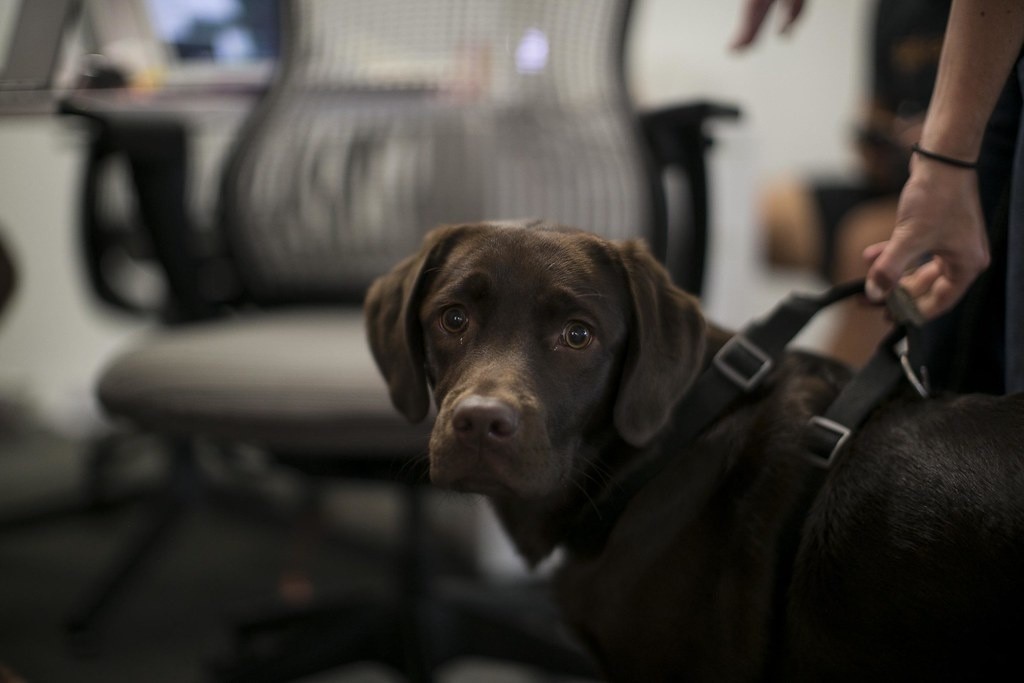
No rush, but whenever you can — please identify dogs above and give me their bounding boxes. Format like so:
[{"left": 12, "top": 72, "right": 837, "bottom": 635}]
[{"left": 364, "top": 218, "right": 1024, "bottom": 683}]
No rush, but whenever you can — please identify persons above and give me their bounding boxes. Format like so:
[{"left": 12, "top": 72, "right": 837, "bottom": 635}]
[{"left": 727, "top": 0, "right": 1024, "bottom": 398}]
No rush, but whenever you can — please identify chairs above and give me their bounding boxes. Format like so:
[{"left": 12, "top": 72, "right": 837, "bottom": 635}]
[{"left": 59, "top": 0, "right": 742, "bottom": 683}]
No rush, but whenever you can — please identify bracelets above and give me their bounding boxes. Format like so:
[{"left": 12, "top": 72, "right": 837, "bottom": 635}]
[{"left": 913, "top": 141, "right": 979, "bottom": 170}]
